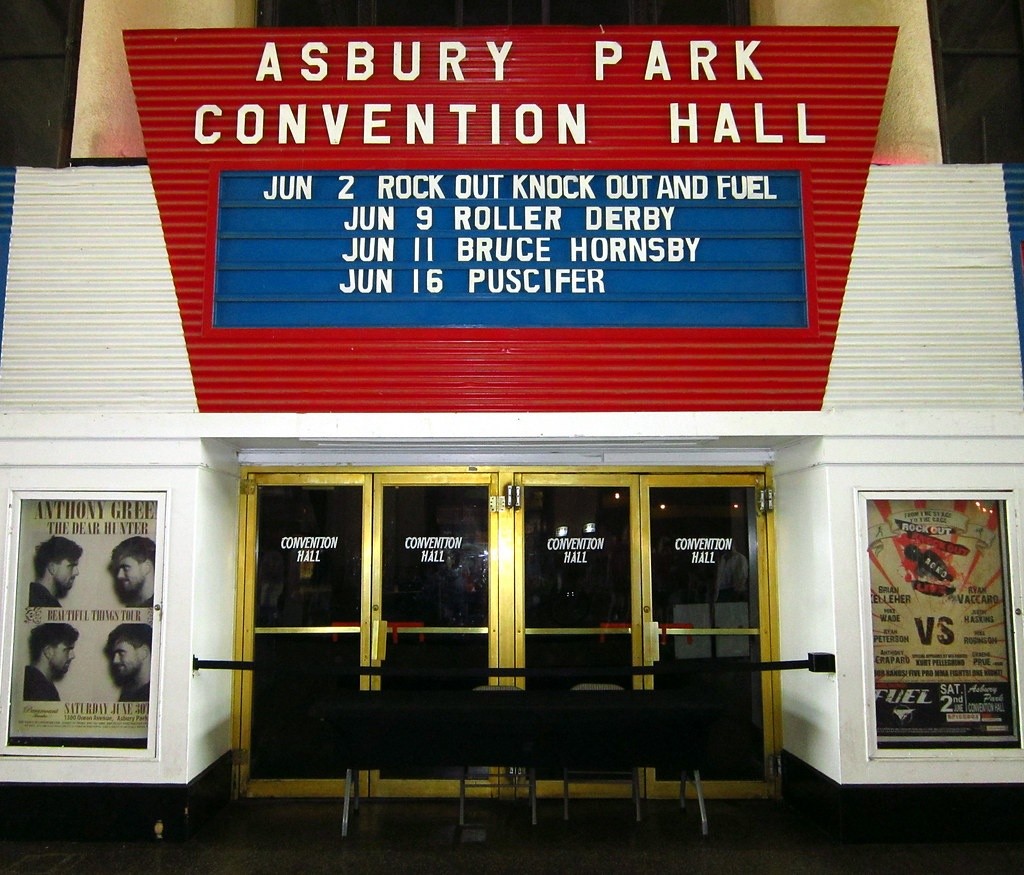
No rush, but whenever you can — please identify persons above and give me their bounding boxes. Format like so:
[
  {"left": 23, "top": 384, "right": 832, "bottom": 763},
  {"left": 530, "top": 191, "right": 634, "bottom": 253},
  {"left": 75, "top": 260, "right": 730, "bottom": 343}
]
[
  {"left": 29, "top": 535, "right": 83, "bottom": 607},
  {"left": 111, "top": 536, "right": 156, "bottom": 607},
  {"left": 435, "top": 548, "right": 469, "bottom": 635},
  {"left": 589, "top": 526, "right": 748, "bottom": 642},
  {"left": 108, "top": 624, "right": 153, "bottom": 702},
  {"left": 23, "top": 623, "right": 79, "bottom": 702}
]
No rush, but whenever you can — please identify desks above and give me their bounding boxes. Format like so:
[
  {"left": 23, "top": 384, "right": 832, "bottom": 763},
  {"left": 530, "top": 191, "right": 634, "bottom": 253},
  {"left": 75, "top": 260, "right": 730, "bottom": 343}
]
[{"left": 314, "top": 690, "right": 719, "bottom": 836}]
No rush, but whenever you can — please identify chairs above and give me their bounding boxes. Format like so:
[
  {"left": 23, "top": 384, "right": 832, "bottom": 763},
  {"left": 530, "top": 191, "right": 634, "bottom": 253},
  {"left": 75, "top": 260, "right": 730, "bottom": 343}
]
[
  {"left": 458, "top": 686, "right": 538, "bottom": 828},
  {"left": 563, "top": 683, "right": 642, "bottom": 826}
]
